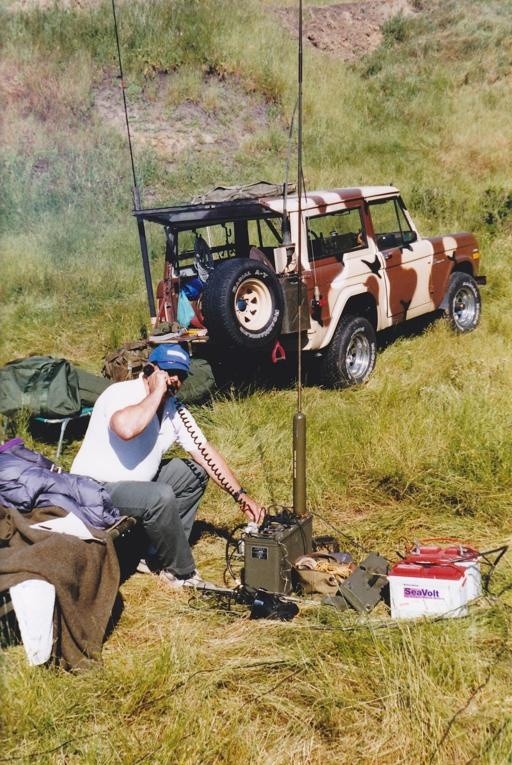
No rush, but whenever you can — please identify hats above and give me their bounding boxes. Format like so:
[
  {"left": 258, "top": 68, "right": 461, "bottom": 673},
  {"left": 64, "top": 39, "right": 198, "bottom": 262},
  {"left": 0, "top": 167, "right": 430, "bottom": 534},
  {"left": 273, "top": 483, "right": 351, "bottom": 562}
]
[{"left": 147, "top": 343, "right": 195, "bottom": 377}]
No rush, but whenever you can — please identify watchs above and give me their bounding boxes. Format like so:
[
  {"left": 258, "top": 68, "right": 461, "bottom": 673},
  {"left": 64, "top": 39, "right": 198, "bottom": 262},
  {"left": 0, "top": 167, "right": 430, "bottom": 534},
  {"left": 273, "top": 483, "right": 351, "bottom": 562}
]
[{"left": 231, "top": 487, "right": 250, "bottom": 499}]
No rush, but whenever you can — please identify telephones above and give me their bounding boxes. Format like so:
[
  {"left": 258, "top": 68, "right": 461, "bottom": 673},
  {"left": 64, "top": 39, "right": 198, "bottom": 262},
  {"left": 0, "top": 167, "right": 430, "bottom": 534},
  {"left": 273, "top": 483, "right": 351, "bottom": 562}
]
[{"left": 144, "top": 365, "right": 175, "bottom": 392}]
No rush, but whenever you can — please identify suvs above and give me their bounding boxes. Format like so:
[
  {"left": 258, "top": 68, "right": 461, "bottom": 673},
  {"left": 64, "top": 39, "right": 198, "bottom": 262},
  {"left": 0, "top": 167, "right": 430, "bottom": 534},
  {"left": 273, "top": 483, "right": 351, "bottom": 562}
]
[{"left": 132, "top": 185, "right": 487, "bottom": 390}]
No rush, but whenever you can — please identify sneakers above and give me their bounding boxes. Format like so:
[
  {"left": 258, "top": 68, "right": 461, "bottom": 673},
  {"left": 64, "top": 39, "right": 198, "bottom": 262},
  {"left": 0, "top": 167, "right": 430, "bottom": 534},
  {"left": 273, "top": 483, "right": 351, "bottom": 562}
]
[{"left": 134, "top": 556, "right": 217, "bottom": 593}]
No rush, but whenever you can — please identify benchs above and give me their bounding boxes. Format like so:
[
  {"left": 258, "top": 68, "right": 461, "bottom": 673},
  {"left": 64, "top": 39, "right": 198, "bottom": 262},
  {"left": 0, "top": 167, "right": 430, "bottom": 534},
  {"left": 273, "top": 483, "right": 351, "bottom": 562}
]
[{"left": 1, "top": 514, "right": 141, "bottom": 622}]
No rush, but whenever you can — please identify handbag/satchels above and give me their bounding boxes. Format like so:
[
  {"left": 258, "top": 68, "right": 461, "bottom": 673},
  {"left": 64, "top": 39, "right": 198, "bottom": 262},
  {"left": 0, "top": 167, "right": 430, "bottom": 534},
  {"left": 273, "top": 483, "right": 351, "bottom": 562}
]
[
  {"left": 0, "top": 355, "right": 83, "bottom": 420},
  {"left": 289, "top": 549, "right": 357, "bottom": 598}
]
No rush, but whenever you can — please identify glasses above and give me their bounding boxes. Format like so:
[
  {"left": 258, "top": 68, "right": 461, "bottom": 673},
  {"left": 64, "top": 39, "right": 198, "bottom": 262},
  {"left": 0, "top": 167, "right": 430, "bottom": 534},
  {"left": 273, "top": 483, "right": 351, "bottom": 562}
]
[{"left": 167, "top": 369, "right": 189, "bottom": 382}]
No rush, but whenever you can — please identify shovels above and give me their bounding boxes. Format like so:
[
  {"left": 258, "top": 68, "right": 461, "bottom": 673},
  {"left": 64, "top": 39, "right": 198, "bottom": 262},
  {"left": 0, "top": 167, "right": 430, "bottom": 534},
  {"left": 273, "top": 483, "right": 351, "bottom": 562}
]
[{"left": 249, "top": 246, "right": 286, "bottom": 363}]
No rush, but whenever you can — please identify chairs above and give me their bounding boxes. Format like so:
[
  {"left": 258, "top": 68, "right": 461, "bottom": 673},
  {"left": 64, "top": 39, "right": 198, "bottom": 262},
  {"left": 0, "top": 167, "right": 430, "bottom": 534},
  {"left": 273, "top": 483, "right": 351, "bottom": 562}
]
[{"left": 20, "top": 357, "right": 94, "bottom": 462}]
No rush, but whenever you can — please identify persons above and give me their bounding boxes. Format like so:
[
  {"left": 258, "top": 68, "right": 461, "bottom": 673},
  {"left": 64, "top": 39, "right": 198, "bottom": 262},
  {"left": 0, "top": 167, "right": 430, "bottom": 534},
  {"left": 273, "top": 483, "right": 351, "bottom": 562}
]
[{"left": 69, "top": 342, "right": 266, "bottom": 591}]
